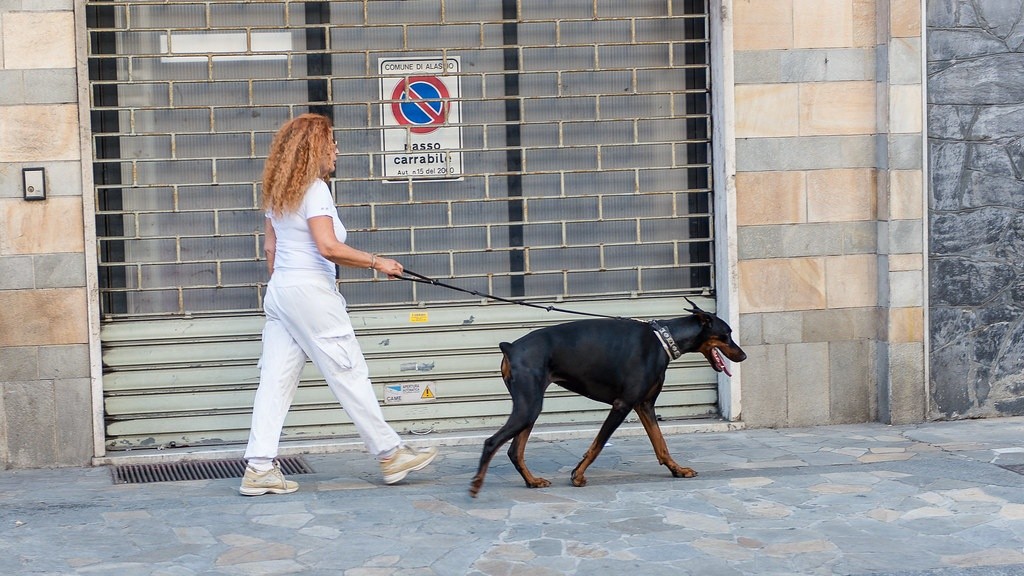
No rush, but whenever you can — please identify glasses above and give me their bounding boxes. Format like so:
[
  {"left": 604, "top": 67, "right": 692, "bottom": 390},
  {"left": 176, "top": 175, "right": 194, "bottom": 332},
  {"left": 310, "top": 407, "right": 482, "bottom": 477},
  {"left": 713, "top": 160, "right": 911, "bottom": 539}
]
[{"left": 330, "top": 140, "right": 337, "bottom": 147}]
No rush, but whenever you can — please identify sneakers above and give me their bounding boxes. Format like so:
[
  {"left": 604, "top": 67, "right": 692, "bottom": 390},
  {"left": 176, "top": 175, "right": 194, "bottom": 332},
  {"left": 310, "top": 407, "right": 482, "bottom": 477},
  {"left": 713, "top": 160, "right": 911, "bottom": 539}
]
[
  {"left": 239, "top": 464, "right": 299, "bottom": 495},
  {"left": 377, "top": 445, "right": 437, "bottom": 484}
]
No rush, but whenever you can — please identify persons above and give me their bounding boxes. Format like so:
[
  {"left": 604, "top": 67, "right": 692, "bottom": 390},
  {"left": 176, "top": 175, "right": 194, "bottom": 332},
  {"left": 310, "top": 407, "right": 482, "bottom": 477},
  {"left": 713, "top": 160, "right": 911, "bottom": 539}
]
[{"left": 237, "top": 113, "right": 438, "bottom": 498}]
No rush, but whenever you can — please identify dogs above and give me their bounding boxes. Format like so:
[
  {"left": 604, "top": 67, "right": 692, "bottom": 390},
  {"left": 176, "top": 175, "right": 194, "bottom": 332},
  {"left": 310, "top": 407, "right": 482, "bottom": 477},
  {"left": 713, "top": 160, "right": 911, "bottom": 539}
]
[{"left": 467, "top": 294, "right": 747, "bottom": 499}]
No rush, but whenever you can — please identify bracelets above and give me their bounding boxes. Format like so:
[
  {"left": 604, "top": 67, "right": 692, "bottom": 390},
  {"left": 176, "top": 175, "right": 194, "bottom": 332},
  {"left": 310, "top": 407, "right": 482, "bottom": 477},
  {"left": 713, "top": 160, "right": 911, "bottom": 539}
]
[{"left": 368, "top": 254, "right": 376, "bottom": 271}]
